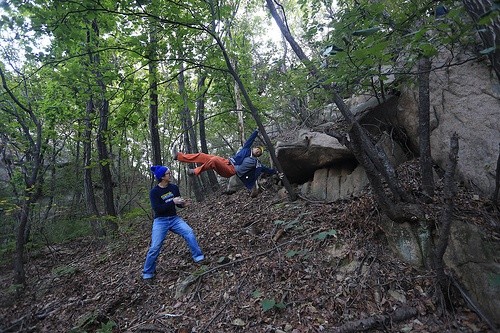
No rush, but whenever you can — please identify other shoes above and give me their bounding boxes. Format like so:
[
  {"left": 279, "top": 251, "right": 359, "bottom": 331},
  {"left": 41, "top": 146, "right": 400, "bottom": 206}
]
[
  {"left": 143, "top": 277, "right": 160, "bottom": 285},
  {"left": 172, "top": 145, "right": 178, "bottom": 160},
  {"left": 188, "top": 168, "right": 194, "bottom": 176},
  {"left": 194, "top": 260, "right": 209, "bottom": 265}
]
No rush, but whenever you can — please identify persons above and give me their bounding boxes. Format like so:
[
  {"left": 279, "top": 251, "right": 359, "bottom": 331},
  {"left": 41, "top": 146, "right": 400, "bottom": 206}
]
[
  {"left": 174, "top": 128, "right": 285, "bottom": 193},
  {"left": 141, "top": 166, "right": 210, "bottom": 285}
]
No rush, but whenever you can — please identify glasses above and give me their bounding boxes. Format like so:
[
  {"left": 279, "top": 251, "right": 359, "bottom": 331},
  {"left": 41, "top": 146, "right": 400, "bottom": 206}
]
[{"left": 165, "top": 172, "right": 173, "bottom": 176}]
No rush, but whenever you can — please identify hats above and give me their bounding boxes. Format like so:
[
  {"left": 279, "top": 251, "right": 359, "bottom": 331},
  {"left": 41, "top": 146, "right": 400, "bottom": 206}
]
[
  {"left": 151, "top": 165, "right": 170, "bottom": 182},
  {"left": 256, "top": 146, "right": 263, "bottom": 154}
]
